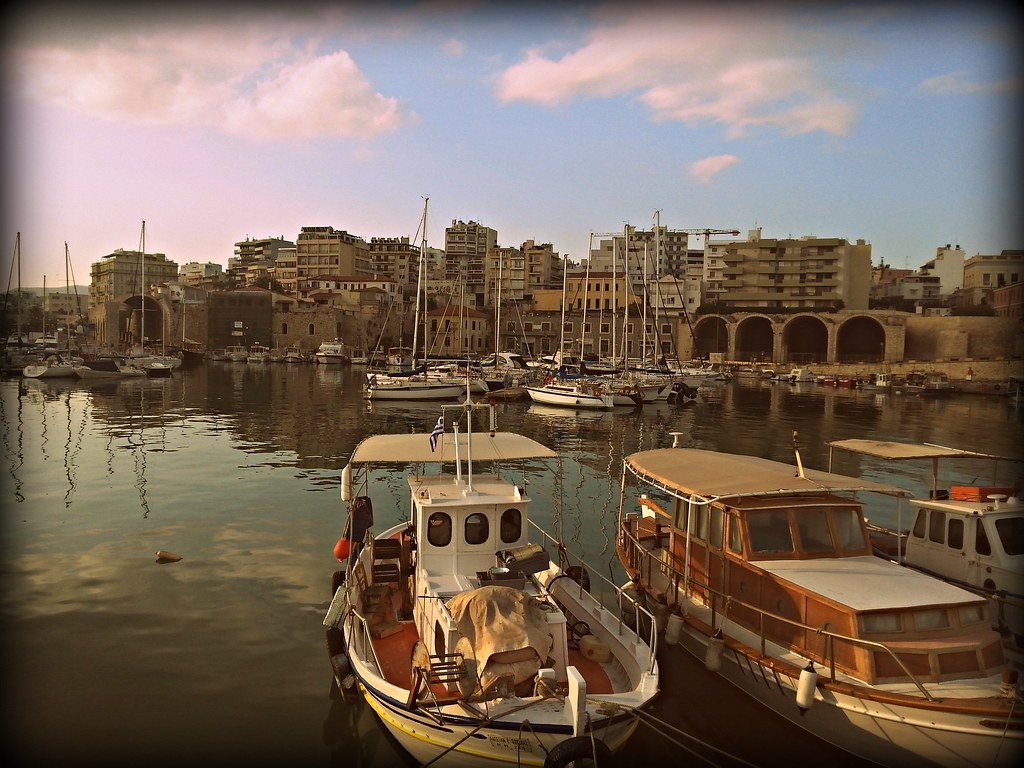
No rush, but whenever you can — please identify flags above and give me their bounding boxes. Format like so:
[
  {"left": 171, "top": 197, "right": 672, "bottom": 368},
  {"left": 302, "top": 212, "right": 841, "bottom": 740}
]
[{"left": 430, "top": 410, "right": 444, "bottom": 452}]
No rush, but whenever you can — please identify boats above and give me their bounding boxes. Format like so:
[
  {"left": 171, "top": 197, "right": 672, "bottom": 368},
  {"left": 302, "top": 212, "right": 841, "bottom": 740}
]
[
  {"left": 316, "top": 337, "right": 345, "bottom": 364},
  {"left": 823, "top": 438, "right": 1023, "bottom": 670},
  {"left": 526, "top": 380, "right": 614, "bottom": 409},
  {"left": 212, "top": 342, "right": 303, "bottom": 365},
  {"left": 322, "top": 367, "right": 659, "bottom": 768},
  {"left": 350, "top": 346, "right": 369, "bottom": 364},
  {"left": 617, "top": 432, "right": 1024, "bottom": 768},
  {"left": 696, "top": 363, "right": 955, "bottom": 395},
  {"left": 72, "top": 366, "right": 146, "bottom": 379}
]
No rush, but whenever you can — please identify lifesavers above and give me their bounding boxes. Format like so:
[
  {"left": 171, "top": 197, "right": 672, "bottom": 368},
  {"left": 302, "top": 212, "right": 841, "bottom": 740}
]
[
  {"left": 331, "top": 569, "right": 346, "bottom": 598},
  {"left": 564, "top": 565, "right": 591, "bottom": 594},
  {"left": 330, "top": 654, "right": 363, "bottom": 707},
  {"left": 623, "top": 386, "right": 631, "bottom": 395},
  {"left": 324, "top": 628, "right": 345, "bottom": 655}
]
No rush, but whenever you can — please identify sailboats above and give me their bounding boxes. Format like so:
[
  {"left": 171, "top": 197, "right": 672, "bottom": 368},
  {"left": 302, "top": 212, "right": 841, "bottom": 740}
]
[
  {"left": 554, "top": 211, "right": 699, "bottom": 404},
  {"left": 406, "top": 252, "right": 530, "bottom": 382},
  {"left": 111, "top": 221, "right": 181, "bottom": 376},
  {"left": 0, "top": 232, "right": 85, "bottom": 378},
  {"left": 169, "top": 288, "right": 205, "bottom": 362},
  {"left": 358, "top": 194, "right": 464, "bottom": 402}
]
